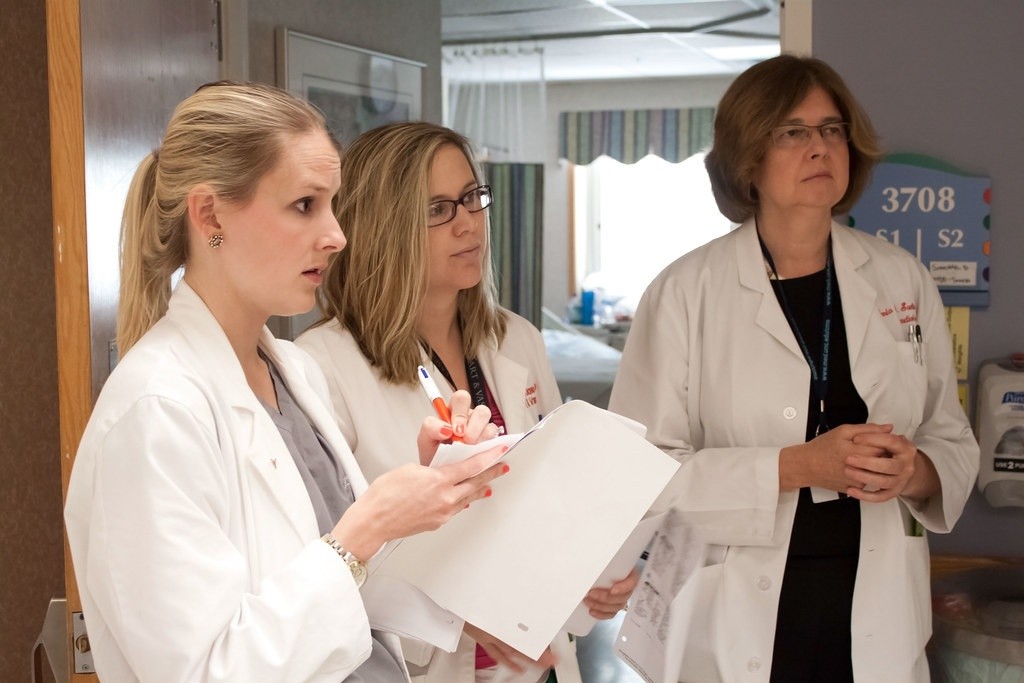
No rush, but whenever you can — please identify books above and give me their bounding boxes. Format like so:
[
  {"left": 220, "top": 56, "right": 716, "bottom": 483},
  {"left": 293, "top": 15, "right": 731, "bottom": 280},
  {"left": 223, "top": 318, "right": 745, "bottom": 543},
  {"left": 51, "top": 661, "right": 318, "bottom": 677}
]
[{"left": 360, "top": 399, "right": 682, "bottom": 660}]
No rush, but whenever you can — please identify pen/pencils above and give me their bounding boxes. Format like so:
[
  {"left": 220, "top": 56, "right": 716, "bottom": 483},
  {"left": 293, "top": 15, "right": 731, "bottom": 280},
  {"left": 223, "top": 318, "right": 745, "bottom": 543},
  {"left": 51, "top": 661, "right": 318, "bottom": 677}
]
[
  {"left": 908, "top": 323, "right": 924, "bottom": 367},
  {"left": 418, "top": 364, "right": 464, "bottom": 444}
]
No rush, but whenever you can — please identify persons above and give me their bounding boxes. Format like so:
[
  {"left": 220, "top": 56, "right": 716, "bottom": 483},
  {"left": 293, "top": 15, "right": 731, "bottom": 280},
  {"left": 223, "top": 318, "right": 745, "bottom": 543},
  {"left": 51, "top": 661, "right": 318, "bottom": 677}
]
[
  {"left": 605, "top": 55, "right": 979, "bottom": 683},
  {"left": 64, "top": 80, "right": 638, "bottom": 683}
]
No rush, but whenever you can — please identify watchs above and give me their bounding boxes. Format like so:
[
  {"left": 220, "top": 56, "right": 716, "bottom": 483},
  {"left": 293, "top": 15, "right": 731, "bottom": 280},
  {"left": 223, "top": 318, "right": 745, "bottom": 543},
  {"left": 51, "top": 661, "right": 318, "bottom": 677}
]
[{"left": 320, "top": 533, "right": 368, "bottom": 590}]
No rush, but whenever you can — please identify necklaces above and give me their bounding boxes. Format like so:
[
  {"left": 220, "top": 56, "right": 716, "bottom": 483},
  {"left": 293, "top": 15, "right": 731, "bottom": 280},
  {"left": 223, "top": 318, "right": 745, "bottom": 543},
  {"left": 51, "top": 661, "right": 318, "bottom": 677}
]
[{"left": 420, "top": 308, "right": 492, "bottom": 423}]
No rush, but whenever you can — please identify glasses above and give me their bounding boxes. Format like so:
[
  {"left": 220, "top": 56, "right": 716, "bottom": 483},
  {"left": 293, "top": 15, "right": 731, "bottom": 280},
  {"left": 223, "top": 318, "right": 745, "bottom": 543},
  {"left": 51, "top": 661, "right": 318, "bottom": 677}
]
[
  {"left": 763, "top": 119, "right": 852, "bottom": 148},
  {"left": 421, "top": 184, "right": 493, "bottom": 227}
]
